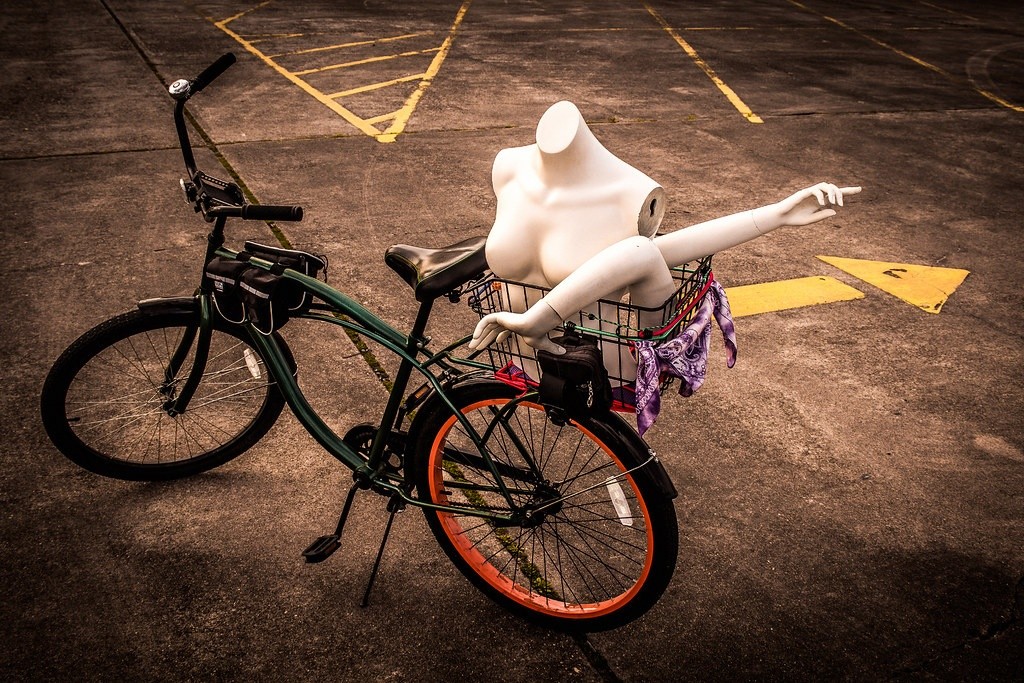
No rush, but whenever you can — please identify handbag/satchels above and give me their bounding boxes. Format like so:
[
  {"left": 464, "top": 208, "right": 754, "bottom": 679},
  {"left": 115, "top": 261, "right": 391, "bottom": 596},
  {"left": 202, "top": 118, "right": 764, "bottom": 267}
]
[{"left": 537, "top": 333, "right": 615, "bottom": 422}]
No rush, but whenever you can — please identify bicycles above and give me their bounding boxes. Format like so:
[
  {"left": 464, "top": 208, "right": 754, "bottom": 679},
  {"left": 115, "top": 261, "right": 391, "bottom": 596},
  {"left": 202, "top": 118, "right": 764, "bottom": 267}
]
[{"left": 42, "top": 52, "right": 678, "bottom": 632}]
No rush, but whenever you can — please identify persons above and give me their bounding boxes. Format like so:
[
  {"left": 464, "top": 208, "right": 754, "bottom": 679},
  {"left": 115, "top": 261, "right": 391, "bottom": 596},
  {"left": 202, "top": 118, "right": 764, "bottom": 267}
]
[{"left": 469, "top": 100, "right": 862, "bottom": 389}]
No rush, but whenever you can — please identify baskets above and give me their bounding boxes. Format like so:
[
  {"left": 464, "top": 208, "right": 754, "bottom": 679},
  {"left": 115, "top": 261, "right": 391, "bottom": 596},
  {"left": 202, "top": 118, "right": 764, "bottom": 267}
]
[{"left": 467, "top": 235, "right": 711, "bottom": 407}]
